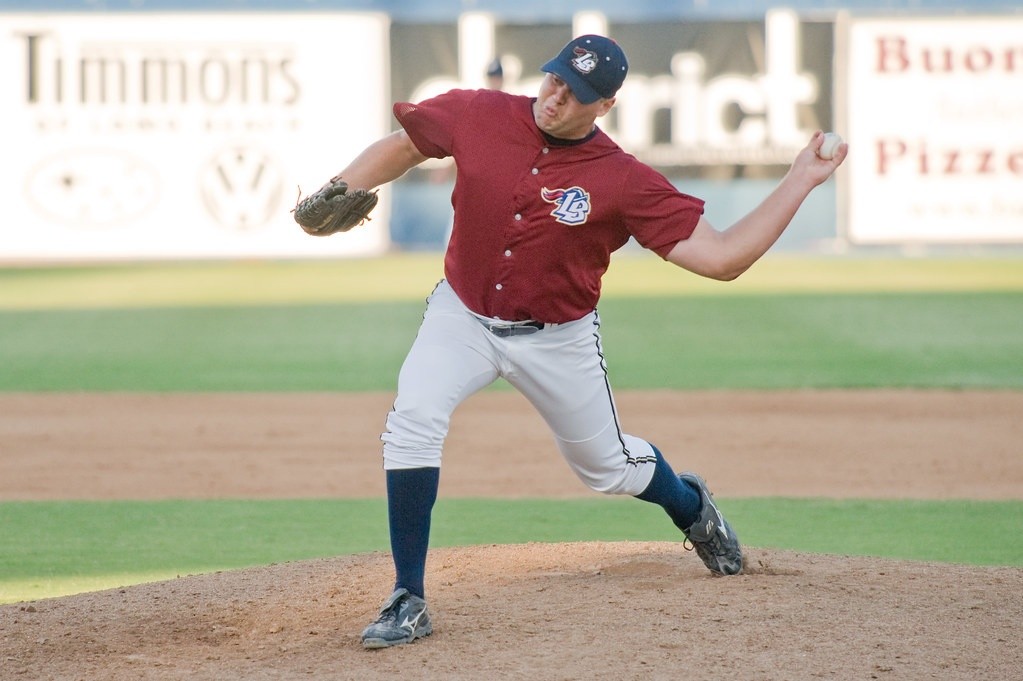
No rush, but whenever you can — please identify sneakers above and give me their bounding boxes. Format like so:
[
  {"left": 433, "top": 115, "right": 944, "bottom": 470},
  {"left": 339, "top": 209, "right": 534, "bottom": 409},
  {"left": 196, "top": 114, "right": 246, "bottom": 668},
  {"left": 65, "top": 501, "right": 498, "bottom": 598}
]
[
  {"left": 676, "top": 471, "right": 743, "bottom": 577},
  {"left": 361, "top": 587, "right": 433, "bottom": 648}
]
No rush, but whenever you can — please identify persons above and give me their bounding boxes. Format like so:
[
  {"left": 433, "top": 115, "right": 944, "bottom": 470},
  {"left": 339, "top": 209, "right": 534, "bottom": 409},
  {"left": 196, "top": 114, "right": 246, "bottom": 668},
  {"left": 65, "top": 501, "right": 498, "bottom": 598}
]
[{"left": 296, "top": 34, "right": 849, "bottom": 647}]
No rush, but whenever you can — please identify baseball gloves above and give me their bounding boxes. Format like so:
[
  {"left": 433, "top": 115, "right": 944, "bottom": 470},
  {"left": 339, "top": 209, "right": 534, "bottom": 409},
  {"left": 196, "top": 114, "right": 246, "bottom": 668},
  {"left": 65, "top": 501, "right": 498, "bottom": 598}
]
[{"left": 289, "top": 174, "right": 381, "bottom": 238}]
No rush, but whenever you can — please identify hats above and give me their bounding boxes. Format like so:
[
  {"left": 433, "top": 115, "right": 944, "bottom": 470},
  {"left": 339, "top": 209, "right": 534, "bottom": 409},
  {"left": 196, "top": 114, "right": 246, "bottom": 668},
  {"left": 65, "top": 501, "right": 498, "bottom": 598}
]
[{"left": 541, "top": 35, "right": 628, "bottom": 103}]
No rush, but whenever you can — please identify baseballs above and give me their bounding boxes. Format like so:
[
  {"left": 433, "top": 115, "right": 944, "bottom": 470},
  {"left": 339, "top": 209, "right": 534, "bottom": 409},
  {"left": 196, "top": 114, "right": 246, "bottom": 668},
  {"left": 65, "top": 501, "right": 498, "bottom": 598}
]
[{"left": 816, "top": 132, "right": 843, "bottom": 160}]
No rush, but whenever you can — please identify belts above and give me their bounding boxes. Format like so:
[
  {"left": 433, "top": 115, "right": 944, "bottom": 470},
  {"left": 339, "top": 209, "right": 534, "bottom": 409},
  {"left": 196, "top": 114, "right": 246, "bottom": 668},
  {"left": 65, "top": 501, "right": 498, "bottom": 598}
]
[{"left": 476, "top": 316, "right": 563, "bottom": 337}]
